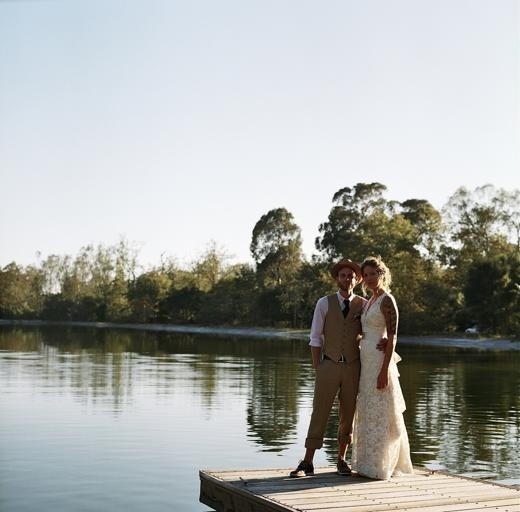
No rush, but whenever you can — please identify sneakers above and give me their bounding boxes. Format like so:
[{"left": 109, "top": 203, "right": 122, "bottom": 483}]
[
  {"left": 290, "top": 461, "right": 314, "bottom": 477},
  {"left": 337, "top": 460, "right": 351, "bottom": 475}
]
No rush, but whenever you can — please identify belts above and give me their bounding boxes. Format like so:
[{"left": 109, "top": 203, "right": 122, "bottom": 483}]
[{"left": 322, "top": 354, "right": 346, "bottom": 362}]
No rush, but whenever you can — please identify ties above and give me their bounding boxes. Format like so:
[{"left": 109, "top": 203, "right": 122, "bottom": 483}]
[{"left": 342, "top": 300, "right": 349, "bottom": 318}]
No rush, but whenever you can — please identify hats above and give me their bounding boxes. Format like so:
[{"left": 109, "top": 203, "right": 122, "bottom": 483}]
[{"left": 331, "top": 259, "right": 363, "bottom": 285}]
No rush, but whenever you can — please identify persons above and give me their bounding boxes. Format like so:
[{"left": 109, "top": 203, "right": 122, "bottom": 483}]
[
  {"left": 349, "top": 255, "right": 414, "bottom": 480},
  {"left": 288, "top": 258, "right": 389, "bottom": 478}
]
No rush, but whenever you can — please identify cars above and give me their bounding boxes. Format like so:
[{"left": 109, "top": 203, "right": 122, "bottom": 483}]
[{"left": 464, "top": 324, "right": 478, "bottom": 333}]
